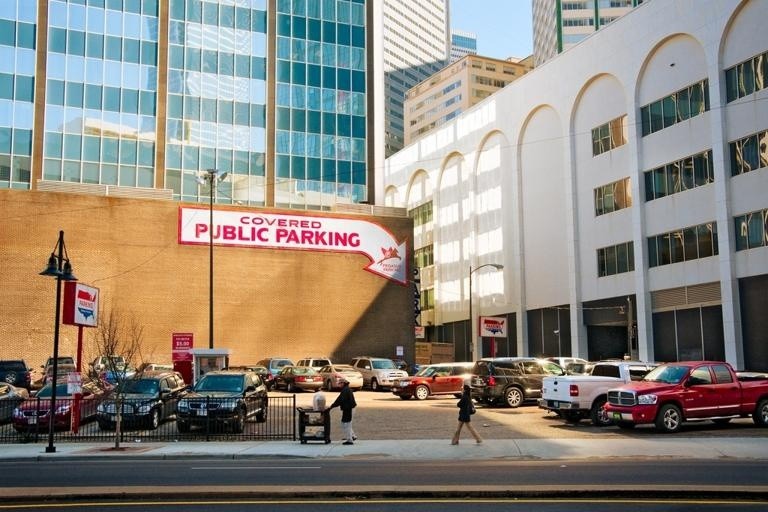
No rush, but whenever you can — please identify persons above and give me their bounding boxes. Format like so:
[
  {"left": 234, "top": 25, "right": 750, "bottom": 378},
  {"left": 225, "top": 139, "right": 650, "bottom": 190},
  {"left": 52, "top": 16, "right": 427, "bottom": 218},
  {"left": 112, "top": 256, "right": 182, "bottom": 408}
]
[
  {"left": 451, "top": 384, "right": 483, "bottom": 445},
  {"left": 328, "top": 378, "right": 358, "bottom": 446}
]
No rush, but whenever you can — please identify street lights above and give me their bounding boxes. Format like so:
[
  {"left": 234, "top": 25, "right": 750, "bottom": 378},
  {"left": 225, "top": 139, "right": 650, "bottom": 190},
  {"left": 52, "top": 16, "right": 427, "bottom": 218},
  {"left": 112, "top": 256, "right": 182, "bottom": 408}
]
[
  {"left": 38, "top": 230, "right": 79, "bottom": 452},
  {"left": 202, "top": 167, "right": 230, "bottom": 348},
  {"left": 469, "top": 263, "right": 504, "bottom": 362}
]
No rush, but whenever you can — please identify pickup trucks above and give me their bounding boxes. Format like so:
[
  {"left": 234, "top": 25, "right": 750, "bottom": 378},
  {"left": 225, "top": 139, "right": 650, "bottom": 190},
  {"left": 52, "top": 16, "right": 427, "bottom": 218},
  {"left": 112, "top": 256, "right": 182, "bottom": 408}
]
[
  {"left": 601, "top": 362, "right": 768, "bottom": 433},
  {"left": 542, "top": 362, "right": 660, "bottom": 426}
]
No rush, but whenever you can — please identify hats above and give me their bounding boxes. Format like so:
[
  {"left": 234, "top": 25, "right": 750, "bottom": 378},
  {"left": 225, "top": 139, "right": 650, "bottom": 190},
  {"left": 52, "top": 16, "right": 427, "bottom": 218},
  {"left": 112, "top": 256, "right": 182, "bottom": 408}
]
[{"left": 338, "top": 379, "right": 347, "bottom": 383}]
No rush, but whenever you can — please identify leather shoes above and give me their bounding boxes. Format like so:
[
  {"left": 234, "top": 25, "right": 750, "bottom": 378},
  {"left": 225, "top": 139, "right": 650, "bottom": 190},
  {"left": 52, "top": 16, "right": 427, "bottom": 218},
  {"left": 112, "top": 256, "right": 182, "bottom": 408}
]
[{"left": 343, "top": 442, "right": 353, "bottom": 444}]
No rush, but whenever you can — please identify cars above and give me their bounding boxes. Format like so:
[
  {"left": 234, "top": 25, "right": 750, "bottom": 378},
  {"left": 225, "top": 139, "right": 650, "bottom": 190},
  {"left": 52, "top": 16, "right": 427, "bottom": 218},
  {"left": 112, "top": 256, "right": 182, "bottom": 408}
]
[
  {"left": 407, "top": 364, "right": 420, "bottom": 375},
  {"left": 42, "top": 364, "right": 77, "bottom": 384},
  {"left": 274, "top": 366, "right": 324, "bottom": 392},
  {"left": 318, "top": 364, "right": 363, "bottom": 391},
  {"left": 12, "top": 376, "right": 117, "bottom": 432},
  {"left": 0, "top": 381, "right": 29, "bottom": 424},
  {"left": 221, "top": 365, "right": 274, "bottom": 392},
  {"left": 96, "top": 370, "right": 187, "bottom": 429},
  {"left": 143, "top": 363, "right": 174, "bottom": 371},
  {"left": 392, "top": 358, "right": 409, "bottom": 370}
]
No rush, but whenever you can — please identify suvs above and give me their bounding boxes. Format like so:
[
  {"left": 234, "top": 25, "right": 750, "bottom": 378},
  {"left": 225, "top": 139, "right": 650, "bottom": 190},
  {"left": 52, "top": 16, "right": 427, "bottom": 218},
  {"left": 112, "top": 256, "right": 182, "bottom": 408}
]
[
  {"left": 256, "top": 358, "right": 295, "bottom": 375},
  {"left": 88, "top": 356, "right": 126, "bottom": 377},
  {"left": 296, "top": 358, "right": 331, "bottom": 371},
  {"left": 173, "top": 371, "right": 268, "bottom": 433},
  {"left": 40, "top": 355, "right": 76, "bottom": 372},
  {"left": 0, "top": 360, "right": 33, "bottom": 392},
  {"left": 350, "top": 357, "right": 409, "bottom": 391},
  {"left": 391, "top": 362, "right": 472, "bottom": 400},
  {"left": 471, "top": 358, "right": 576, "bottom": 407},
  {"left": 544, "top": 356, "right": 589, "bottom": 369}
]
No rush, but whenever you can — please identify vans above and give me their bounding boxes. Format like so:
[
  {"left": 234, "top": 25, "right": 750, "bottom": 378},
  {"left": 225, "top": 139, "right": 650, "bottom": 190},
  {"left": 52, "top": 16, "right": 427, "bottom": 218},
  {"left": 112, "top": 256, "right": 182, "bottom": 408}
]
[{"left": 564, "top": 362, "right": 595, "bottom": 375}]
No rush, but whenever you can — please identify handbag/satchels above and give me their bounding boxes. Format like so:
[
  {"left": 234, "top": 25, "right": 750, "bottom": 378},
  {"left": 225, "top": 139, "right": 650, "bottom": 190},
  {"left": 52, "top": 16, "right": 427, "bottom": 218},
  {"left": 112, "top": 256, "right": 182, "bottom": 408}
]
[{"left": 471, "top": 397, "right": 476, "bottom": 415}]
